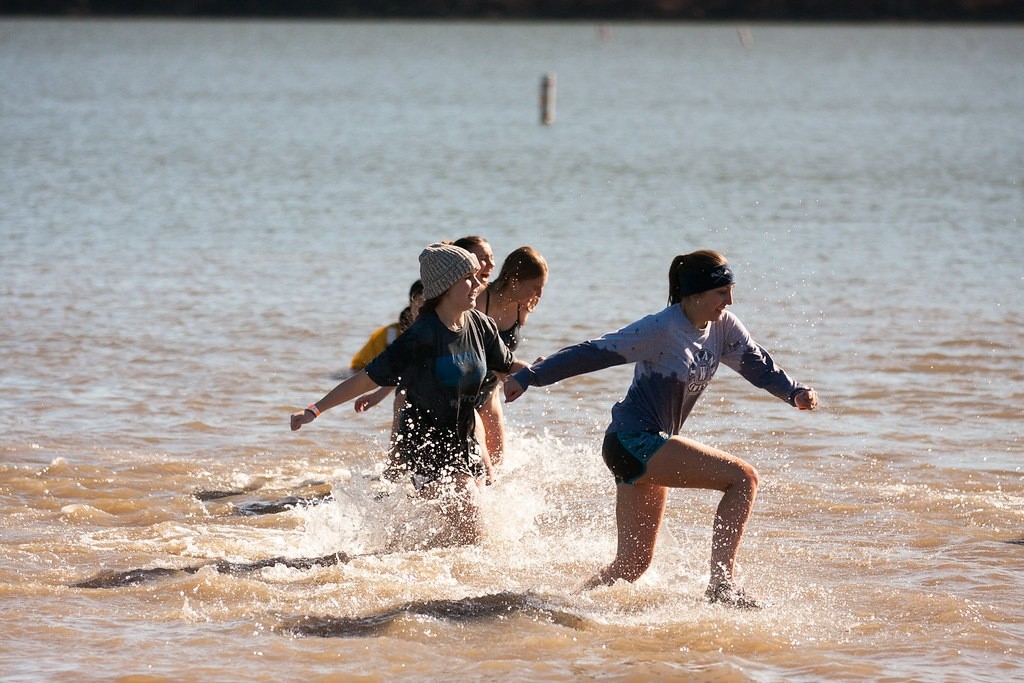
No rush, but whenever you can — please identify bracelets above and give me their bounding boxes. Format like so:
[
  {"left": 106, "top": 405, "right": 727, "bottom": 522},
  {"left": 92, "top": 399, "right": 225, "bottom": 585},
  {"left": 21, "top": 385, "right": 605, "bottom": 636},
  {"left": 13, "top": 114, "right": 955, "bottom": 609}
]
[
  {"left": 305, "top": 409, "right": 317, "bottom": 418},
  {"left": 308, "top": 404, "right": 321, "bottom": 416}
]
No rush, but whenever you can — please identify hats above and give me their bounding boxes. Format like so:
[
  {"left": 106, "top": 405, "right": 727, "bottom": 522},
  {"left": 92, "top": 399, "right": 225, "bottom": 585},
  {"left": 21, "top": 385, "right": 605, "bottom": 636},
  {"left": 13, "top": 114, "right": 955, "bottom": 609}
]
[{"left": 418, "top": 243, "right": 481, "bottom": 299}]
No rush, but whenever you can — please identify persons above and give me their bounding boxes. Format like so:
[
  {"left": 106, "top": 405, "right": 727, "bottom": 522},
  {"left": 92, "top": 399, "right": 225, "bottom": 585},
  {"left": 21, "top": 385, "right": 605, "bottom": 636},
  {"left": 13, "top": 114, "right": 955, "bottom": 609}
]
[
  {"left": 503, "top": 249, "right": 819, "bottom": 611},
  {"left": 291, "top": 236, "right": 549, "bottom": 552}
]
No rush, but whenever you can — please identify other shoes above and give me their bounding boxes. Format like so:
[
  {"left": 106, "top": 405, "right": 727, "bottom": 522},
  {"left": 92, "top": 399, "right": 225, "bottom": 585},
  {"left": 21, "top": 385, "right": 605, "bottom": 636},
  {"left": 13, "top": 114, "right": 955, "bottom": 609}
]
[{"left": 707, "top": 582, "right": 766, "bottom": 614}]
